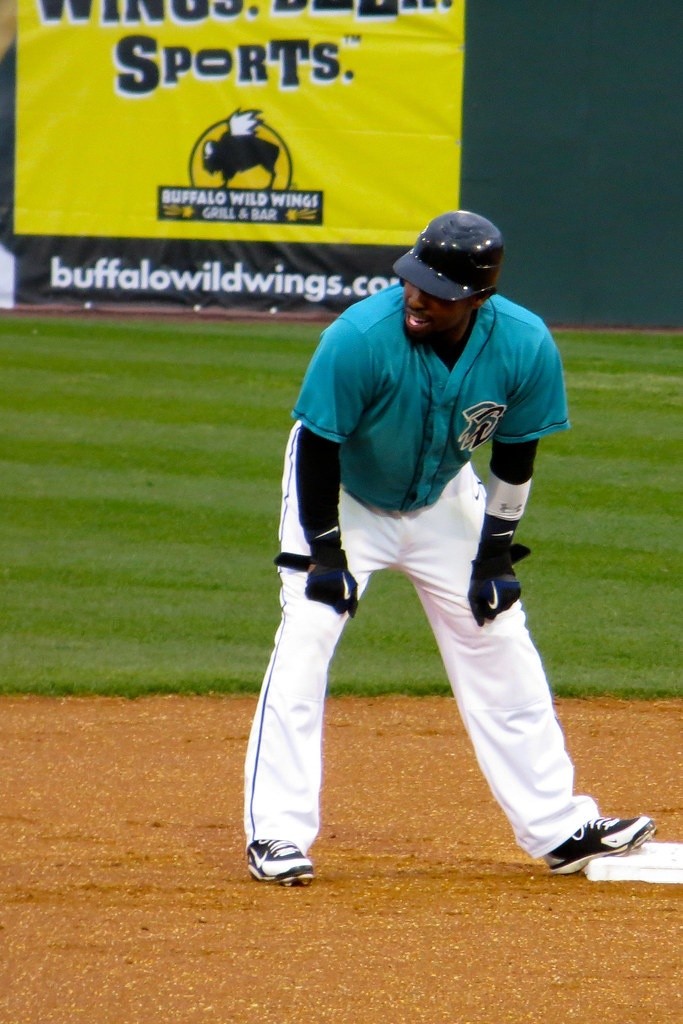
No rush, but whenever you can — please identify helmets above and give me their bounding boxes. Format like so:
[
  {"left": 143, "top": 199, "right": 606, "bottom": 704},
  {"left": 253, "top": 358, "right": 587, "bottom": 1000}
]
[{"left": 394, "top": 211, "right": 506, "bottom": 301}]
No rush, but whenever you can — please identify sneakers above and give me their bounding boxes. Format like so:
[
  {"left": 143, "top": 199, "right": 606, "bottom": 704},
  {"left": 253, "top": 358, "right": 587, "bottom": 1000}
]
[
  {"left": 246, "top": 839, "right": 316, "bottom": 887},
  {"left": 543, "top": 815, "right": 657, "bottom": 875}
]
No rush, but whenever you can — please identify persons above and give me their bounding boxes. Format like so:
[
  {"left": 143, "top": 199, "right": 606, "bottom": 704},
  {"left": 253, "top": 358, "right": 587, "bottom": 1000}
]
[{"left": 245, "top": 210, "right": 654, "bottom": 885}]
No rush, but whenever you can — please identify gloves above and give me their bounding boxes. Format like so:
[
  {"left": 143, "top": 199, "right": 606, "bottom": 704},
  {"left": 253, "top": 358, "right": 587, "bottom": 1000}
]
[
  {"left": 274, "top": 549, "right": 360, "bottom": 617},
  {"left": 468, "top": 542, "right": 532, "bottom": 623}
]
